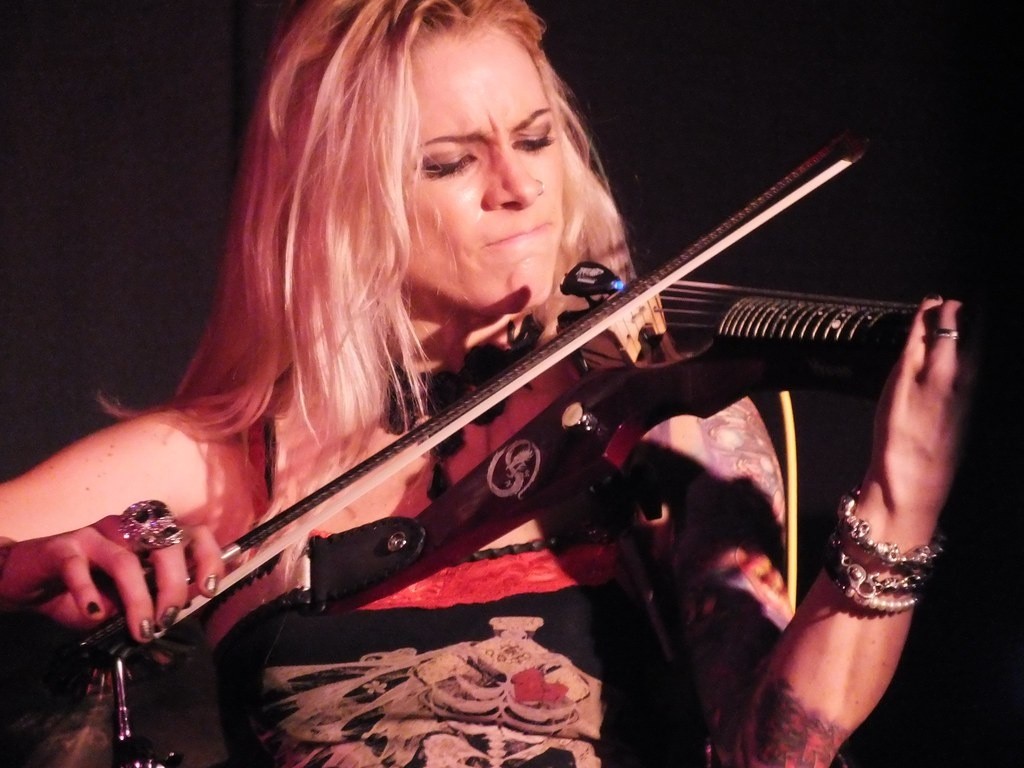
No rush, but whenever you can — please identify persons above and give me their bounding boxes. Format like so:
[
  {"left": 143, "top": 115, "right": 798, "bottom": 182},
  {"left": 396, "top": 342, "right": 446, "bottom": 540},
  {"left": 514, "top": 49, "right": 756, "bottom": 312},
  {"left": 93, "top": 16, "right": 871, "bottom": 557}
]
[{"left": 3, "top": 0, "right": 1012, "bottom": 768}]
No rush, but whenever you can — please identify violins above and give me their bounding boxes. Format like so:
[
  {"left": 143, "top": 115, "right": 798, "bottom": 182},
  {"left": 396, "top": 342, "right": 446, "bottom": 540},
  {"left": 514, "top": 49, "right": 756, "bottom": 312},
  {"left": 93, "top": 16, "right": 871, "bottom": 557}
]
[{"left": 553, "top": 259, "right": 922, "bottom": 422}]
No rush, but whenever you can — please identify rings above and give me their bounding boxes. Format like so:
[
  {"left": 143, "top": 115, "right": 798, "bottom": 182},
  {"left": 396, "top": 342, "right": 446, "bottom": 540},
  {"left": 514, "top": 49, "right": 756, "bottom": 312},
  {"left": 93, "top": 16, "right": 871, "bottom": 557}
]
[
  {"left": 121, "top": 500, "right": 186, "bottom": 556},
  {"left": 932, "top": 323, "right": 962, "bottom": 344}
]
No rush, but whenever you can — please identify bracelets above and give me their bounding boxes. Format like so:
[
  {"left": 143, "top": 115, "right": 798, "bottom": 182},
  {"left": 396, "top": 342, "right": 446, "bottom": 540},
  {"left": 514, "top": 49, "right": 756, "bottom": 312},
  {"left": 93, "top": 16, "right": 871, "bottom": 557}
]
[
  {"left": 835, "top": 482, "right": 951, "bottom": 569},
  {"left": 815, "top": 526, "right": 929, "bottom": 616}
]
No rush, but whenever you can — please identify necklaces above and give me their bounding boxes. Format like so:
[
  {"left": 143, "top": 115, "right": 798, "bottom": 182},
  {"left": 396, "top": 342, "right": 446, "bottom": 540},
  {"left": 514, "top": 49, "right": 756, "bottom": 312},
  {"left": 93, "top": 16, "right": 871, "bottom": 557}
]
[{"left": 384, "top": 358, "right": 440, "bottom": 432}]
